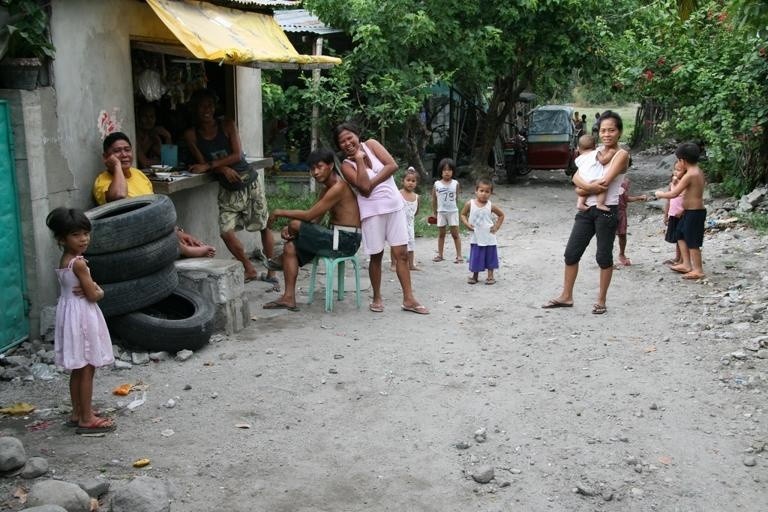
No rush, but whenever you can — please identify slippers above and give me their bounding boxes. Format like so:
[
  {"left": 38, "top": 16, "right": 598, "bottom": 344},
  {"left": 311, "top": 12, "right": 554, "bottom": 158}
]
[
  {"left": 591, "top": 303, "right": 606, "bottom": 314},
  {"left": 370, "top": 301, "right": 383, "bottom": 313},
  {"left": 241, "top": 272, "right": 282, "bottom": 292},
  {"left": 263, "top": 254, "right": 284, "bottom": 273},
  {"left": 261, "top": 300, "right": 301, "bottom": 313},
  {"left": 619, "top": 254, "right": 631, "bottom": 267},
  {"left": 399, "top": 303, "right": 430, "bottom": 315},
  {"left": 540, "top": 298, "right": 575, "bottom": 309},
  {"left": 75, "top": 417, "right": 118, "bottom": 434},
  {"left": 433, "top": 255, "right": 465, "bottom": 263},
  {"left": 663, "top": 256, "right": 706, "bottom": 281},
  {"left": 467, "top": 275, "right": 497, "bottom": 286},
  {"left": 69, "top": 407, "right": 107, "bottom": 424}
]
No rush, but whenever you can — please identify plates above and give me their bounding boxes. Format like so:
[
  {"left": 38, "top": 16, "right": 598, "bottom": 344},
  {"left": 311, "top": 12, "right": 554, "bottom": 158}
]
[{"left": 150, "top": 164, "right": 172, "bottom": 179}]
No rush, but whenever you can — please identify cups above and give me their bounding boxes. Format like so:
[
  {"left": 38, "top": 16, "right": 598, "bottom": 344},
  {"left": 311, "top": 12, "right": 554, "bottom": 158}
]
[{"left": 161, "top": 144, "right": 177, "bottom": 169}]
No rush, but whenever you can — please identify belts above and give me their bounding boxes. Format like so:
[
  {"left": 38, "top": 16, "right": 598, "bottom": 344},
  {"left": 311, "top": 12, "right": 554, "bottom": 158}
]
[{"left": 329, "top": 224, "right": 362, "bottom": 253}]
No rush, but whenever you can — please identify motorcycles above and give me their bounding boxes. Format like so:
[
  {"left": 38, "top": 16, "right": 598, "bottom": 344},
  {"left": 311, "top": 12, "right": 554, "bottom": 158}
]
[{"left": 502, "top": 103, "right": 578, "bottom": 186}]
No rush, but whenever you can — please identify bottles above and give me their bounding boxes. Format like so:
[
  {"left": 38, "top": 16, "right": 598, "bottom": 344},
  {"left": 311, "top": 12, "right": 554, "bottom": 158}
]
[{"left": 428, "top": 217, "right": 437, "bottom": 224}]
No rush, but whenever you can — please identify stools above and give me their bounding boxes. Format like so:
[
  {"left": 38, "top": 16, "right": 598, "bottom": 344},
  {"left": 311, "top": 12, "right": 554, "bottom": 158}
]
[{"left": 306, "top": 255, "right": 361, "bottom": 313}]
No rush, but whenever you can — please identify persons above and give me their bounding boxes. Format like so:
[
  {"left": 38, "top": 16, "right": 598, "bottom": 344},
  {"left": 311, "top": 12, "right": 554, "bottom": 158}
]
[
  {"left": 429, "top": 156, "right": 464, "bottom": 264},
  {"left": 388, "top": 164, "right": 421, "bottom": 275},
  {"left": 44, "top": 206, "right": 117, "bottom": 433},
  {"left": 572, "top": 134, "right": 615, "bottom": 212},
  {"left": 594, "top": 112, "right": 600, "bottom": 124},
  {"left": 541, "top": 107, "right": 630, "bottom": 316},
  {"left": 590, "top": 123, "right": 598, "bottom": 149},
  {"left": 459, "top": 177, "right": 504, "bottom": 287},
  {"left": 662, "top": 160, "right": 685, "bottom": 266},
  {"left": 182, "top": 89, "right": 279, "bottom": 283},
  {"left": 580, "top": 114, "right": 587, "bottom": 134},
  {"left": 259, "top": 147, "right": 362, "bottom": 313},
  {"left": 334, "top": 122, "right": 430, "bottom": 317},
  {"left": 573, "top": 111, "right": 583, "bottom": 132},
  {"left": 652, "top": 142, "right": 707, "bottom": 279},
  {"left": 136, "top": 104, "right": 173, "bottom": 168},
  {"left": 90, "top": 131, "right": 216, "bottom": 259},
  {"left": 613, "top": 179, "right": 646, "bottom": 271}
]
